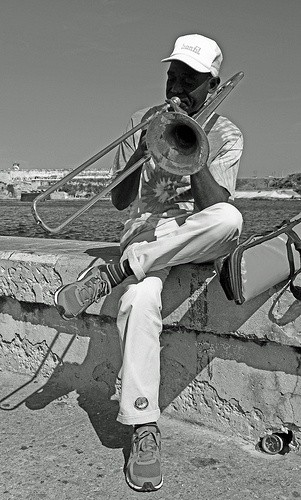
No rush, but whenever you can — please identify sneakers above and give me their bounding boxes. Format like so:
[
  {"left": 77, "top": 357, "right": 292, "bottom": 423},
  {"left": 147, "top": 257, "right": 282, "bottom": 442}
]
[
  {"left": 52, "top": 264, "right": 113, "bottom": 321},
  {"left": 124, "top": 422, "right": 164, "bottom": 491}
]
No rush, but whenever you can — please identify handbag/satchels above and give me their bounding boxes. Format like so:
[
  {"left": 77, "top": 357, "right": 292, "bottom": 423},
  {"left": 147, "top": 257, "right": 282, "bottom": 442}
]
[{"left": 226, "top": 212, "right": 301, "bottom": 306}]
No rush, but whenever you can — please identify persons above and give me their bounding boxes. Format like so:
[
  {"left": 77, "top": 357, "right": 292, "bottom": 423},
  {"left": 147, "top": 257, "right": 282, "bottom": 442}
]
[{"left": 54, "top": 34, "right": 245, "bottom": 491}]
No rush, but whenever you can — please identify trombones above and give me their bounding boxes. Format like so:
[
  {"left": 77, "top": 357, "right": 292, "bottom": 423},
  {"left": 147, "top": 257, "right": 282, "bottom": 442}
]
[{"left": 30, "top": 71, "right": 245, "bottom": 235}]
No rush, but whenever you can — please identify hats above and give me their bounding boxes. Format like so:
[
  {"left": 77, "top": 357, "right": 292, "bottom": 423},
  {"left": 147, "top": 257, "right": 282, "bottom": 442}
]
[{"left": 160, "top": 32, "right": 223, "bottom": 78}]
